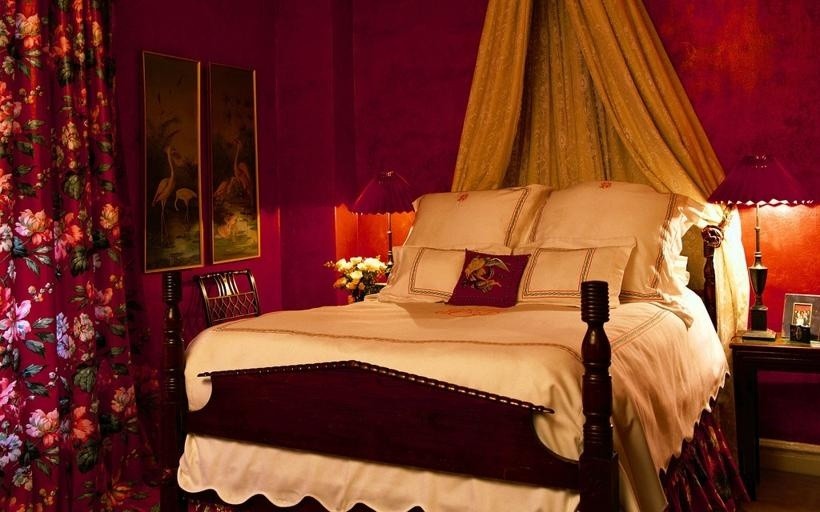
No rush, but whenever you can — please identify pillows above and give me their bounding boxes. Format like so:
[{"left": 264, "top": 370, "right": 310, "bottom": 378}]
[
  {"left": 369, "top": 184, "right": 557, "bottom": 306},
  {"left": 439, "top": 246, "right": 531, "bottom": 309},
  {"left": 512, "top": 236, "right": 639, "bottom": 312},
  {"left": 376, "top": 241, "right": 514, "bottom": 307},
  {"left": 523, "top": 178, "right": 709, "bottom": 333}
]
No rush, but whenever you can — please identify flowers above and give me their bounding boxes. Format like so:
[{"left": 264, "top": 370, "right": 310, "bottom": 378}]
[{"left": 317, "top": 252, "right": 394, "bottom": 306}]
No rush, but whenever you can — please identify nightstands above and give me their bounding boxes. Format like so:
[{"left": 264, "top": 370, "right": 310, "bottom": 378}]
[{"left": 725, "top": 327, "right": 820, "bottom": 501}]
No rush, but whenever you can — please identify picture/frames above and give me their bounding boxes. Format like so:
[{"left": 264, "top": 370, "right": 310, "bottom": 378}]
[
  {"left": 130, "top": 48, "right": 206, "bottom": 277},
  {"left": 779, "top": 292, "right": 820, "bottom": 344},
  {"left": 200, "top": 57, "right": 263, "bottom": 265}
]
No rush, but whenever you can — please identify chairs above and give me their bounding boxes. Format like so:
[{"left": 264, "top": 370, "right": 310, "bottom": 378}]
[{"left": 190, "top": 267, "right": 263, "bottom": 328}]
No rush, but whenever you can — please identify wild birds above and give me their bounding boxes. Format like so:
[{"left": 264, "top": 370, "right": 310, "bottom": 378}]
[
  {"left": 174, "top": 188, "right": 197, "bottom": 222},
  {"left": 152, "top": 145, "right": 176, "bottom": 245},
  {"left": 213, "top": 176, "right": 244, "bottom": 217},
  {"left": 232, "top": 138, "right": 255, "bottom": 219}
]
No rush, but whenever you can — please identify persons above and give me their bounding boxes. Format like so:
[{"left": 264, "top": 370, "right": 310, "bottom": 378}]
[{"left": 793, "top": 311, "right": 810, "bottom": 325}]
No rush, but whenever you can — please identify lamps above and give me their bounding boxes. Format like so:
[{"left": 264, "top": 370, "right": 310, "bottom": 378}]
[
  {"left": 348, "top": 168, "right": 420, "bottom": 283},
  {"left": 702, "top": 149, "right": 817, "bottom": 343}
]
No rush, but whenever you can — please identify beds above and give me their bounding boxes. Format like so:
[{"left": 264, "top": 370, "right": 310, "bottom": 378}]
[{"left": 178, "top": 177, "right": 762, "bottom": 512}]
